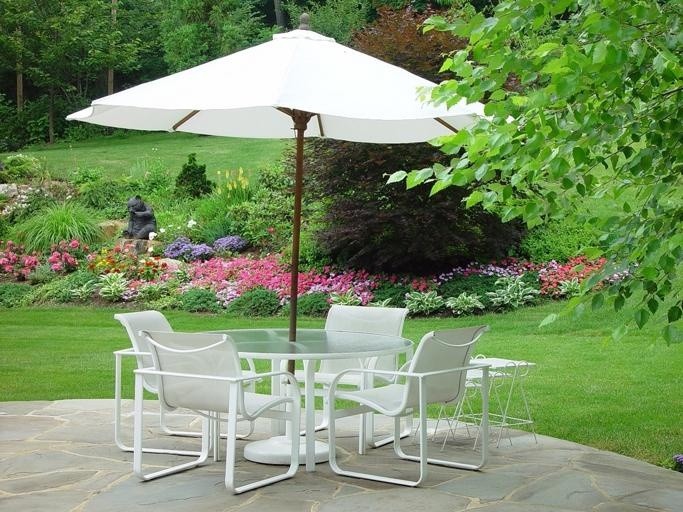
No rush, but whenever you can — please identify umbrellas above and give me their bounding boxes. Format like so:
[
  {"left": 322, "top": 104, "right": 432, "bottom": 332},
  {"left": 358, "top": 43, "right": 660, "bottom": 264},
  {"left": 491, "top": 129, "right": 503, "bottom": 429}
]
[{"left": 65, "top": 12, "right": 515, "bottom": 386}]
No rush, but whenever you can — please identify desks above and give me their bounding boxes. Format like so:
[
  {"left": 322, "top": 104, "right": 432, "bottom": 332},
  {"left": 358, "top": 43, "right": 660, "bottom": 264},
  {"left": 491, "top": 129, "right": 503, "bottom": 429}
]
[{"left": 193, "top": 327, "right": 415, "bottom": 464}]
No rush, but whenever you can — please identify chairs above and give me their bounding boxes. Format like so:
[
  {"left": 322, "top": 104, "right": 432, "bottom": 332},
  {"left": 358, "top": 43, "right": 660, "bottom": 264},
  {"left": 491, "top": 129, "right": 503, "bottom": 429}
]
[
  {"left": 129, "top": 329, "right": 301, "bottom": 497},
  {"left": 112, "top": 310, "right": 254, "bottom": 456},
  {"left": 328, "top": 322, "right": 490, "bottom": 488},
  {"left": 288, "top": 306, "right": 410, "bottom": 453}
]
